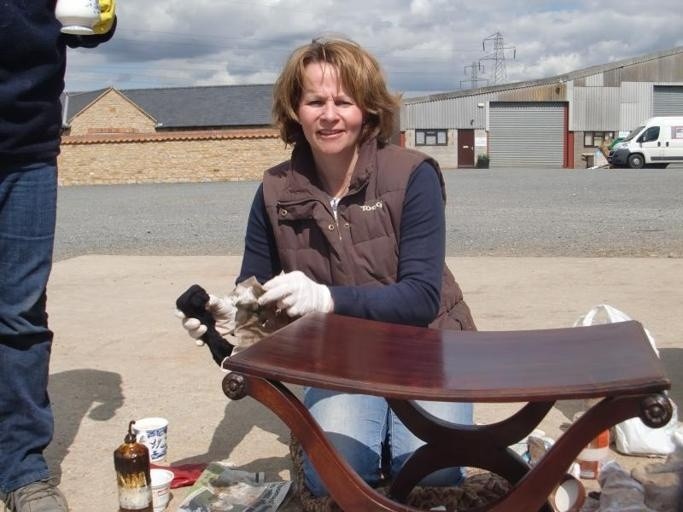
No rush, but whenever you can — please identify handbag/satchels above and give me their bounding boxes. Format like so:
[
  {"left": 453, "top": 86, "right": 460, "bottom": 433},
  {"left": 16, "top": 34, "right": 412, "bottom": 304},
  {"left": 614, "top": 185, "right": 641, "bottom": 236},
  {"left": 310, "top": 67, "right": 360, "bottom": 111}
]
[{"left": 572, "top": 304, "right": 679, "bottom": 458}]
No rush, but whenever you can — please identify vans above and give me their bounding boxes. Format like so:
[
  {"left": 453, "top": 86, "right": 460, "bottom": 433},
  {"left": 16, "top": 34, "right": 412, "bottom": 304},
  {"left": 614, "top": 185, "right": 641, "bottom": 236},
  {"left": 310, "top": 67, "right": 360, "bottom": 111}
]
[{"left": 608, "top": 115, "right": 682, "bottom": 169}]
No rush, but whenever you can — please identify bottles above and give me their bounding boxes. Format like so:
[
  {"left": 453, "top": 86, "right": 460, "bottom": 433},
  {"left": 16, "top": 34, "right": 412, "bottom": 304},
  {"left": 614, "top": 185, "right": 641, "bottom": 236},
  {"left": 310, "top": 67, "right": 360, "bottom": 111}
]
[{"left": 111, "top": 419, "right": 154, "bottom": 512}]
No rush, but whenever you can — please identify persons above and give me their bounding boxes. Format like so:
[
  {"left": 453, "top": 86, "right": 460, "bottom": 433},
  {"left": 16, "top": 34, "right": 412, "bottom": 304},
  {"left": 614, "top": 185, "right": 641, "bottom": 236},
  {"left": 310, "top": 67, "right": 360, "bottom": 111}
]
[
  {"left": 170, "top": 28, "right": 478, "bottom": 498},
  {"left": 1, "top": 0, "right": 119, "bottom": 512}
]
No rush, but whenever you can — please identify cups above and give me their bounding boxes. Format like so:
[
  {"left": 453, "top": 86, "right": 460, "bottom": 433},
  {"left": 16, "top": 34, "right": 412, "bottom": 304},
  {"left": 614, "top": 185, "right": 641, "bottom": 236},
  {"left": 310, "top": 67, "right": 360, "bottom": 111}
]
[
  {"left": 52, "top": 0, "right": 103, "bottom": 35},
  {"left": 549, "top": 474, "right": 586, "bottom": 512},
  {"left": 133, "top": 416, "right": 176, "bottom": 511}
]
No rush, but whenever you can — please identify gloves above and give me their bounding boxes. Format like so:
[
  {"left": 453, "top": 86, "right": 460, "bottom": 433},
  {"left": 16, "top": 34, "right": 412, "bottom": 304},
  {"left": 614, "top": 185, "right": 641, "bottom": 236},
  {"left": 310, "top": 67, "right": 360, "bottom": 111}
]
[
  {"left": 72, "top": 0, "right": 119, "bottom": 42},
  {"left": 257, "top": 269, "right": 331, "bottom": 318},
  {"left": 173, "top": 285, "right": 250, "bottom": 348}
]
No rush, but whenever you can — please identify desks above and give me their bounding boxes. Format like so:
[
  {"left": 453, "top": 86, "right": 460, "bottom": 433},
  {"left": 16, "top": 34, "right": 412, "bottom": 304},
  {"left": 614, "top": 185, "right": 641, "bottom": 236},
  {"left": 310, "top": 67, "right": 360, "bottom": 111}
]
[{"left": 211, "top": 311, "right": 677, "bottom": 511}]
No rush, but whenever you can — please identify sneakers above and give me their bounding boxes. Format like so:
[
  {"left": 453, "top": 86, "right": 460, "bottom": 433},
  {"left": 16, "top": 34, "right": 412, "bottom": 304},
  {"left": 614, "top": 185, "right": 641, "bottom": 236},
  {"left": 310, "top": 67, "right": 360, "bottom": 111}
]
[{"left": 0, "top": 475, "right": 70, "bottom": 512}]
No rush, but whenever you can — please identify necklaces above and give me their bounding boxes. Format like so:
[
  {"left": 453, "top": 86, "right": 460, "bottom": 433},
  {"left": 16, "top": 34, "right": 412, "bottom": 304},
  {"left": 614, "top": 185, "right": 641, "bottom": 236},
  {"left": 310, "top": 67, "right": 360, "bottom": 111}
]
[{"left": 317, "top": 177, "right": 351, "bottom": 211}]
